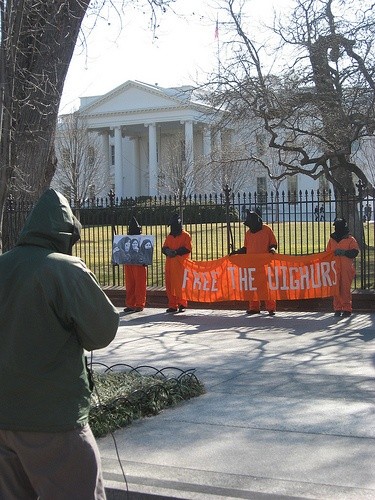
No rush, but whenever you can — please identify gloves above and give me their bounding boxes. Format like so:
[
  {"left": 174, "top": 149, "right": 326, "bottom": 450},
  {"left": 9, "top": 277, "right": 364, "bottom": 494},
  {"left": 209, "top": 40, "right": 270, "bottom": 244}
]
[
  {"left": 269, "top": 247, "right": 275, "bottom": 254},
  {"left": 334, "top": 248, "right": 345, "bottom": 256},
  {"left": 229, "top": 250, "right": 240, "bottom": 256},
  {"left": 142, "top": 263, "right": 147, "bottom": 267},
  {"left": 165, "top": 249, "right": 176, "bottom": 257},
  {"left": 113, "top": 263, "right": 119, "bottom": 266}
]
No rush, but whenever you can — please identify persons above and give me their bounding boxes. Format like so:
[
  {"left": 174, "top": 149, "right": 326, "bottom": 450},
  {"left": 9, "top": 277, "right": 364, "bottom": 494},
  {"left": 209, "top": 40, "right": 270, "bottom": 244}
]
[
  {"left": 0, "top": 189, "right": 119, "bottom": 499},
  {"left": 361, "top": 204, "right": 372, "bottom": 220},
  {"left": 314, "top": 204, "right": 326, "bottom": 221},
  {"left": 327, "top": 218, "right": 360, "bottom": 317},
  {"left": 229, "top": 211, "right": 277, "bottom": 314},
  {"left": 162, "top": 215, "right": 192, "bottom": 312},
  {"left": 124, "top": 218, "right": 148, "bottom": 312}
]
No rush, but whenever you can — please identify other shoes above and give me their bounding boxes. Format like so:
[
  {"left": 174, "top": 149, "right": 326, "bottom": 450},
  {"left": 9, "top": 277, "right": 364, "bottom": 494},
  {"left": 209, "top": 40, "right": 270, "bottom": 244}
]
[
  {"left": 166, "top": 308, "right": 178, "bottom": 312},
  {"left": 136, "top": 307, "right": 143, "bottom": 312},
  {"left": 342, "top": 311, "right": 351, "bottom": 317},
  {"left": 269, "top": 310, "right": 275, "bottom": 315},
  {"left": 246, "top": 310, "right": 260, "bottom": 314},
  {"left": 334, "top": 310, "right": 341, "bottom": 317},
  {"left": 179, "top": 306, "right": 186, "bottom": 312},
  {"left": 124, "top": 307, "right": 134, "bottom": 312}
]
